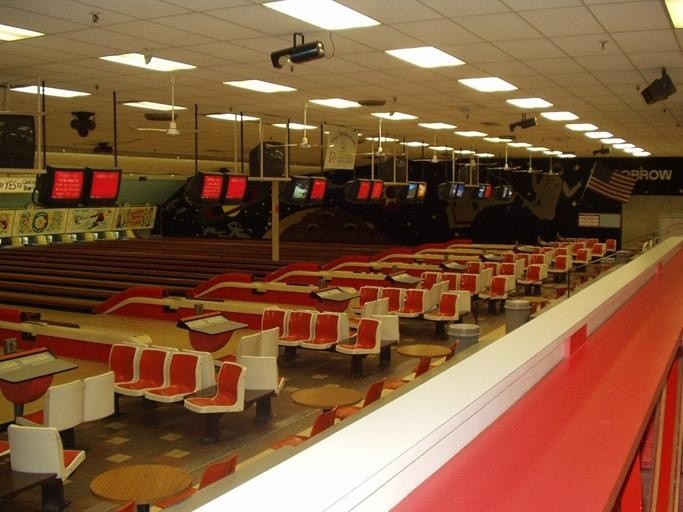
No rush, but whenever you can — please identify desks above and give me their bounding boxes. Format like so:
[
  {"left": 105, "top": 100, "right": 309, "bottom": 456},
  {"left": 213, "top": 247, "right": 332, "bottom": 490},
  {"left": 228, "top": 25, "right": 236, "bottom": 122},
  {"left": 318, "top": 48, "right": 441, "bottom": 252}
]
[
  {"left": 397, "top": 340, "right": 451, "bottom": 382},
  {"left": 91, "top": 464, "right": 193, "bottom": 512},
  {"left": 288, "top": 385, "right": 363, "bottom": 429}
]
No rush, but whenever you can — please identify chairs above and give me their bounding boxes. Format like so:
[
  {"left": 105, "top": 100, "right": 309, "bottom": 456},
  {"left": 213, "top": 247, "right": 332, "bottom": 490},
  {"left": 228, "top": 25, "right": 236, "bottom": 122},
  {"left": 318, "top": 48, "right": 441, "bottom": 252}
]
[
  {"left": 156, "top": 454, "right": 242, "bottom": 511},
  {"left": 348, "top": 285, "right": 460, "bottom": 366},
  {"left": 112, "top": 498, "right": 139, "bottom": 512},
  {"left": 270, "top": 402, "right": 342, "bottom": 452},
  {"left": 216, "top": 325, "right": 288, "bottom": 421},
  {"left": 329, "top": 381, "right": 385, "bottom": 424},
  {"left": 0, "top": 423, "right": 87, "bottom": 508},
  {"left": 410, "top": 340, "right": 461, "bottom": 374},
  {"left": 421, "top": 237, "right": 618, "bottom": 325},
  {"left": 108, "top": 344, "right": 219, "bottom": 419},
  {"left": 383, "top": 354, "right": 433, "bottom": 393},
  {"left": 336, "top": 318, "right": 382, "bottom": 374},
  {"left": 14, "top": 371, "right": 119, "bottom": 447},
  {"left": 183, "top": 360, "right": 250, "bottom": 441},
  {"left": 258, "top": 307, "right": 349, "bottom": 370}
]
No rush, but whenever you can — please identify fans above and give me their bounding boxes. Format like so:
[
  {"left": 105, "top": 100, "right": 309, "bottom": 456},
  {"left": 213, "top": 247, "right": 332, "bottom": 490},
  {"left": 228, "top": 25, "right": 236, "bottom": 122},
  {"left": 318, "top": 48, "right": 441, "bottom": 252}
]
[
  {"left": 267, "top": 96, "right": 336, "bottom": 150},
  {"left": 130, "top": 74, "right": 199, "bottom": 136},
  {"left": 355, "top": 120, "right": 564, "bottom": 178}
]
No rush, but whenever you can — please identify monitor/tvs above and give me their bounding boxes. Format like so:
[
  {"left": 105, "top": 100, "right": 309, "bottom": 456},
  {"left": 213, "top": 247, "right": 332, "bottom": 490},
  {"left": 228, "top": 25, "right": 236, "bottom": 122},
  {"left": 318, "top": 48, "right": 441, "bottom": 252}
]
[
  {"left": 36, "top": 164, "right": 87, "bottom": 208},
  {"left": 0, "top": 114, "right": 35, "bottom": 169},
  {"left": 221, "top": 172, "right": 250, "bottom": 203},
  {"left": 80, "top": 167, "right": 121, "bottom": 207},
  {"left": 249, "top": 141, "right": 284, "bottom": 177},
  {"left": 191, "top": 170, "right": 226, "bottom": 203},
  {"left": 284, "top": 175, "right": 514, "bottom": 204}
]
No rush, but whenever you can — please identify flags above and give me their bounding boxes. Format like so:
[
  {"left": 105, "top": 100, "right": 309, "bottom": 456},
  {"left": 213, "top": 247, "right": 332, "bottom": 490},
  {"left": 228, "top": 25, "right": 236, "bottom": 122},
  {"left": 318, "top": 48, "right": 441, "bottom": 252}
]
[{"left": 589, "top": 158, "right": 638, "bottom": 204}]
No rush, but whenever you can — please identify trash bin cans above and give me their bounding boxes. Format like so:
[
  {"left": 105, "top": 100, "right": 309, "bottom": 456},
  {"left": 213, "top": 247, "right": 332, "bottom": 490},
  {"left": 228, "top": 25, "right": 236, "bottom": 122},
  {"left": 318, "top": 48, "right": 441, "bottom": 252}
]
[
  {"left": 504, "top": 299, "right": 532, "bottom": 335},
  {"left": 447, "top": 323, "right": 481, "bottom": 356}
]
